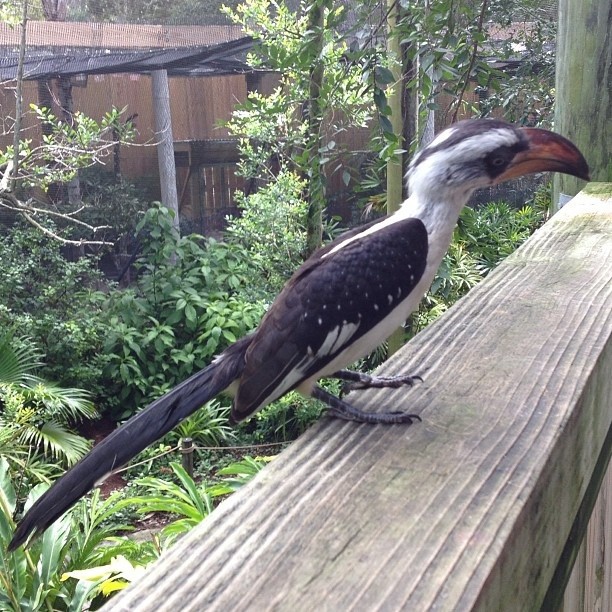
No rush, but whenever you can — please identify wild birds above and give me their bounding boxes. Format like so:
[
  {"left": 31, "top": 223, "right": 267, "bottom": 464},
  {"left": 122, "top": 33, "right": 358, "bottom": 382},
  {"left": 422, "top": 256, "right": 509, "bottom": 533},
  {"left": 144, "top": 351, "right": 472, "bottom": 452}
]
[{"left": 5, "top": 117, "right": 593, "bottom": 558}]
